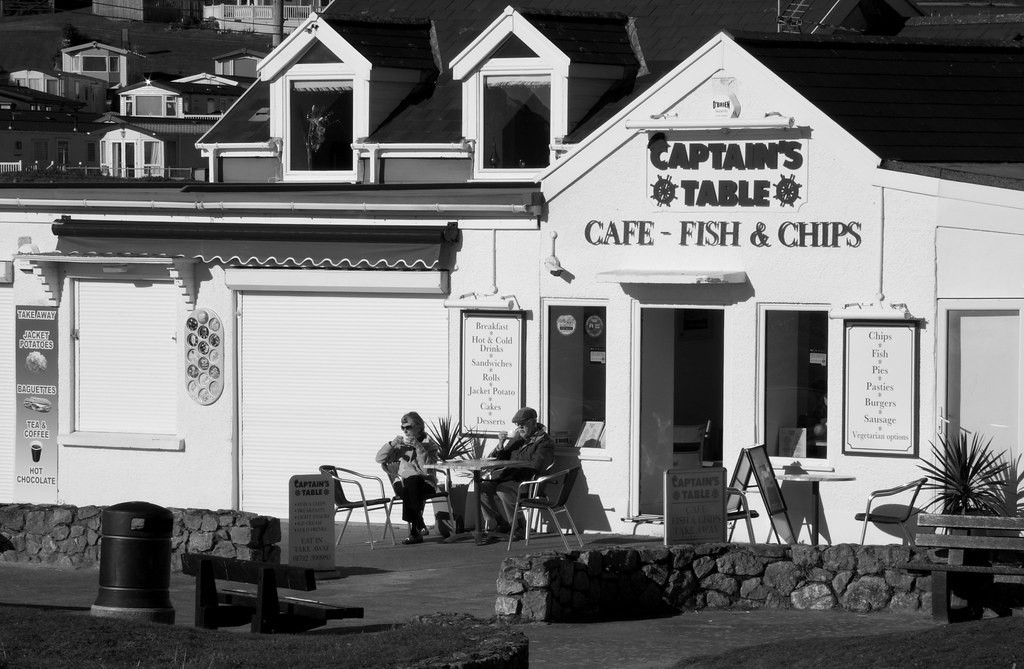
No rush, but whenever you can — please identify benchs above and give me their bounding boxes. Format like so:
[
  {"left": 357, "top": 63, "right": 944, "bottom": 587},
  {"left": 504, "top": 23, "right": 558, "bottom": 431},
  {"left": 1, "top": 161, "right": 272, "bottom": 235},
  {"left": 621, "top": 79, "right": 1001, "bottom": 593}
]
[
  {"left": 899, "top": 514, "right": 1024, "bottom": 625},
  {"left": 180, "top": 553, "right": 365, "bottom": 633}
]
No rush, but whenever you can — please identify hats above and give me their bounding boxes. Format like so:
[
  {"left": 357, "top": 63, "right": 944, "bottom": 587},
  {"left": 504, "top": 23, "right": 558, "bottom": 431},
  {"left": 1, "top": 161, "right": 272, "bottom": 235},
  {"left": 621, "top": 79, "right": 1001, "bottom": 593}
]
[{"left": 512, "top": 407, "right": 538, "bottom": 422}]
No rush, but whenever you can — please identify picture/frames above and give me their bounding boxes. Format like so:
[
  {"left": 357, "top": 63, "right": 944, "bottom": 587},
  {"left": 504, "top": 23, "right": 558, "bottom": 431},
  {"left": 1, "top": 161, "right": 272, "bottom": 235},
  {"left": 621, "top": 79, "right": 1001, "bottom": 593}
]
[
  {"left": 573, "top": 421, "right": 605, "bottom": 448},
  {"left": 842, "top": 318, "right": 920, "bottom": 459},
  {"left": 460, "top": 308, "right": 527, "bottom": 438}
]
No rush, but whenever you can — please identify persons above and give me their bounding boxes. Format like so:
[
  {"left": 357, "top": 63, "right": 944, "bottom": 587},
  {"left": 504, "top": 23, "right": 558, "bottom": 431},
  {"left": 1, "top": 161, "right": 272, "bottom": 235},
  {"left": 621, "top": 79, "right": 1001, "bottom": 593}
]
[
  {"left": 376, "top": 412, "right": 438, "bottom": 545},
  {"left": 479, "top": 406, "right": 555, "bottom": 545}
]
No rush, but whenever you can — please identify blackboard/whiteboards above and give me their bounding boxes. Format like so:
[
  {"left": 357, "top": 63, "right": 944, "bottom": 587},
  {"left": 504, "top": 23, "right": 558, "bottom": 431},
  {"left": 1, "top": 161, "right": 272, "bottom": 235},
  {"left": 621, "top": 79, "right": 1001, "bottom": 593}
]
[{"left": 726, "top": 443, "right": 788, "bottom": 515}]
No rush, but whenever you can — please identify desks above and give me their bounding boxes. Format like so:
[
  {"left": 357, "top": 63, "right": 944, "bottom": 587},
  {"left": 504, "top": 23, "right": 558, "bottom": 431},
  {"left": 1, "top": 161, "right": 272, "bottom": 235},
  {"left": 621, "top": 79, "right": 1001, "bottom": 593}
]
[
  {"left": 775, "top": 472, "right": 856, "bottom": 546},
  {"left": 424, "top": 458, "right": 529, "bottom": 545}
]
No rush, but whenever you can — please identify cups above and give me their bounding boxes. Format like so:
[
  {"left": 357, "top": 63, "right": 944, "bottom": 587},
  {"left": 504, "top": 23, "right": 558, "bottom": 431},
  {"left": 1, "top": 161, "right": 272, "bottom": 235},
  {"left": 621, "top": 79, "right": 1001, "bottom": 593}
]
[
  {"left": 30, "top": 444, "right": 42, "bottom": 462},
  {"left": 402, "top": 436, "right": 412, "bottom": 445},
  {"left": 506, "top": 428, "right": 517, "bottom": 438}
]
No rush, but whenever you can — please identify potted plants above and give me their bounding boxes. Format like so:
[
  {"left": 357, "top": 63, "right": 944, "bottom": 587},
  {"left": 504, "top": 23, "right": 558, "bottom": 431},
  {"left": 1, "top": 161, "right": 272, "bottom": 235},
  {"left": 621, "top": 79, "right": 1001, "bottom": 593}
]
[
  {"left": 423, "top": 416, "right": 477, "bottom": 537},
  {"left": 916, "top": 431, "right": 1008, "bottom": 536}
]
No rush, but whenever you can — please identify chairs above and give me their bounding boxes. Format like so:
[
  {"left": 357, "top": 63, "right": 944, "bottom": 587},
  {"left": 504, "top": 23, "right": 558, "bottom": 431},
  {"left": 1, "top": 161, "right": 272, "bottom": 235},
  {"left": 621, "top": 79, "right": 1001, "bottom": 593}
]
[
  {"left": 671, "top": 420, "right": 711, "bottom": 471},
  {"left": 725, "top": 486, "right": 755, "bottom": 544},
  {"left": 485, "top": 457, "right": 557, "bottom": 533},
  {"left": 319, "top": 464, "right": 397, "bottom": 550},
  {"left": 381, "top": 460, "right": 456, "bottom": 542},
  {"left": 854, "top": 478, "right": 929, "bottom": 545},
  {"left": 507, "top": 466, "right": 584, "bottom": 552}
]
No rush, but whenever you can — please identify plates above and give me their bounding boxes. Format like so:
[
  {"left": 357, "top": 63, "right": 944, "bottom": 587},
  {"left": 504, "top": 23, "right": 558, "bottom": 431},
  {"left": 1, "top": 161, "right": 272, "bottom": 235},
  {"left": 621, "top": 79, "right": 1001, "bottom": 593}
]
[
  {"left": 479, "top": 457, "right": 498, "bottom": 461},
  {"left": 444, "top": 459, "right": 463, "bottom": 464}
]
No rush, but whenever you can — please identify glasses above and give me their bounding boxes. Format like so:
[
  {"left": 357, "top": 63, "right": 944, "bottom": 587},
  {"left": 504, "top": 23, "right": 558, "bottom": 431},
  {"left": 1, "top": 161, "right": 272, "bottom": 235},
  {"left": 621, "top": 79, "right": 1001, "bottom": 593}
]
[
  {"left": 401, "top": 425, "right": 413, "bottom": 431},
  {"left": 515, "top": 423, "right": 529, "bottom": 426}
]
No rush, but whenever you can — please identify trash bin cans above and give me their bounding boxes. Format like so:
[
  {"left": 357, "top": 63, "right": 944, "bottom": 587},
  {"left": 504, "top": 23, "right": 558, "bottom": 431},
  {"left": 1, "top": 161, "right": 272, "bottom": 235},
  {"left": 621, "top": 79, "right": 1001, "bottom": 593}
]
[{"left": 90, "top": 500, "right": 175, "bottom": 624}]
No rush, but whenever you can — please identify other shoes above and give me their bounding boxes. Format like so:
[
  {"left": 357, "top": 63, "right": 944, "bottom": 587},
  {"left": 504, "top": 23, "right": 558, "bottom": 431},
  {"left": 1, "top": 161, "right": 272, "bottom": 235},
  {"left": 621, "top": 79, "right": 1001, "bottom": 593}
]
[
  {"left": 493, "top": 522, "right": 511, "bottom": 534},
  {"left": 419, "top": 526, "right": 430, "bottom": 536},
  {"left": 402, "top": 534, "right": 423, "bottom": 545},
  {"left": 508, "top": 526, "right": 527, "bottom": 541}
]
